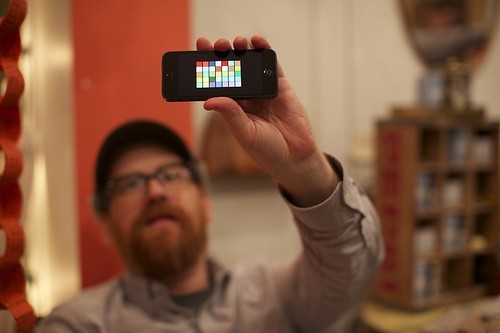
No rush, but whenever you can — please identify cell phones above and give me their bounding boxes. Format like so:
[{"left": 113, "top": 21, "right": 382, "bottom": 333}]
[{"left": 162, "top": 49, "right": 278, "bottom": 102}]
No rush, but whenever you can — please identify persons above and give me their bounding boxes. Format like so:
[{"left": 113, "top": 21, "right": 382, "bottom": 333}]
[{"left": 32, "top": 35, "right": 384, "bottom": 332}]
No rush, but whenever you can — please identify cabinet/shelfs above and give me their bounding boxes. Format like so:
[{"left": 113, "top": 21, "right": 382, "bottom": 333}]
[{"left": 377, "top": 120, "right": 500, "bottom": 313}]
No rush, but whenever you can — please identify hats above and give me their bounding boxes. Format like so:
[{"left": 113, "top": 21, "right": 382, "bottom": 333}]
[{"left": 95, "top": 119, "right": 192, "bottom": 189}]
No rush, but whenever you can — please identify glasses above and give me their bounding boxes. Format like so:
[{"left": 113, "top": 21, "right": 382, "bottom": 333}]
[{"left": 107, "top": 163, "right": 191, "bottom": 206}]
